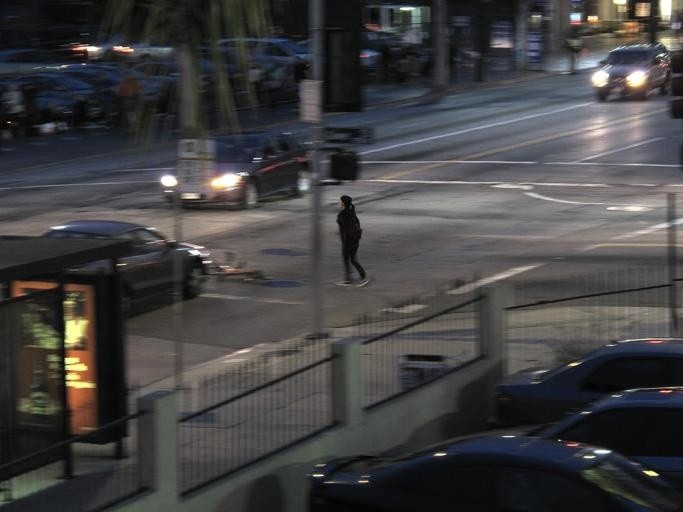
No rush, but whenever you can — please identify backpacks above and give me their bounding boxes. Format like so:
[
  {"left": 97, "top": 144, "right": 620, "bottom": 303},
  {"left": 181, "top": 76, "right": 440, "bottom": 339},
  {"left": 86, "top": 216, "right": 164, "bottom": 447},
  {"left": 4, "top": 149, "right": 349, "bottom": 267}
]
[{"left": 347, "top": 215, "right": 362, "bottom": 240}]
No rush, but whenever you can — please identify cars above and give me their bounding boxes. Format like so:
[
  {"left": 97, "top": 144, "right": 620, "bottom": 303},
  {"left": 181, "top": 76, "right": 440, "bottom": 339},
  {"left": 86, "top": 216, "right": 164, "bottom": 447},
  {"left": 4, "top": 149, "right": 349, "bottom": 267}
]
[
  {"left": 161, "top": 128, "right": 313, "bottom": 209},
  {"left": 39, "top": 217, "right": 211, "bottom": 318}
]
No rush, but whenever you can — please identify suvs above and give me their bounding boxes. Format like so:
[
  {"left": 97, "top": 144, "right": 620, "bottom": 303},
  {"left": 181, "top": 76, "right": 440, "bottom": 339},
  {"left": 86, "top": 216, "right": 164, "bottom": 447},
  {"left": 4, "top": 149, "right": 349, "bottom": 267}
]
[{"left": 590, "top": 41, "right": 670, "bottom": 103}]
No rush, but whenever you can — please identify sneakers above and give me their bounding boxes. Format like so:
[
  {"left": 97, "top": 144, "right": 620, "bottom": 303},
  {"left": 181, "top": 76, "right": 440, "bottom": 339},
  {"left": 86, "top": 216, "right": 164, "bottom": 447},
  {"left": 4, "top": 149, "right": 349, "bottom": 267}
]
[
  {"left": 356, "top": 277, "right": 369, "bottom": 288},
  {"left": 336, "top": 280, "right": 354, "bottom": 286}
]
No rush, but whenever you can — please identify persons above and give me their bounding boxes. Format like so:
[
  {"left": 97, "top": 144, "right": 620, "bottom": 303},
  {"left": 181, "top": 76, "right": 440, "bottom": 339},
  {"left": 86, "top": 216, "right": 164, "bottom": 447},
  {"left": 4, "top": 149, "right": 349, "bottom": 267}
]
[
  {"left": 334, "top": 194, "right": 369, "bottom": 287},
  {"left": 1, "top": 54, "right": 309, "bottom": 140},
  {"left": 376, "top": 25, "right": 584, "bottom": 85}
]
